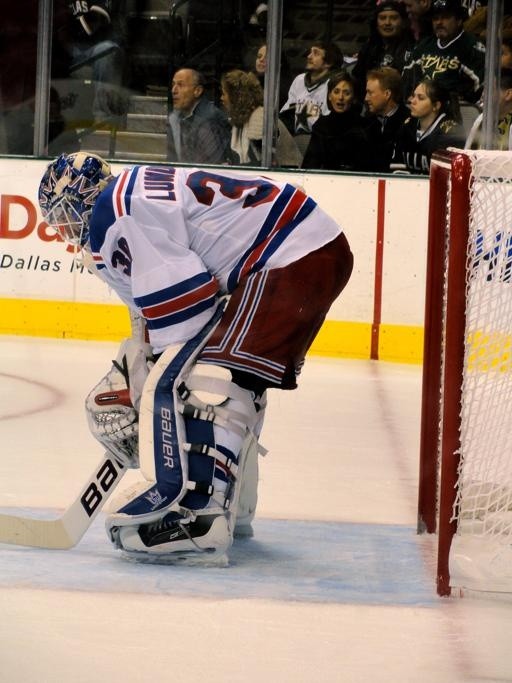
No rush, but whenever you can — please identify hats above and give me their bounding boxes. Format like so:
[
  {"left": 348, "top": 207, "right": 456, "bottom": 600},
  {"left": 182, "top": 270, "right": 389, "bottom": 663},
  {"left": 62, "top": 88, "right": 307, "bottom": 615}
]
[
  {"left": 375, "top": 0, "right": 403, "bottom": 11},
  {"left": 430, "top": 0, "right": 461, "bottom": 17}
]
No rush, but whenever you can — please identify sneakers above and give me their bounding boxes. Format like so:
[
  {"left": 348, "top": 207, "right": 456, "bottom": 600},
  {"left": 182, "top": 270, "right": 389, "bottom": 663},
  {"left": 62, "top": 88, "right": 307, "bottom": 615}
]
[{"left": 114, "top": 511, "right": 231, "bottom": 553}]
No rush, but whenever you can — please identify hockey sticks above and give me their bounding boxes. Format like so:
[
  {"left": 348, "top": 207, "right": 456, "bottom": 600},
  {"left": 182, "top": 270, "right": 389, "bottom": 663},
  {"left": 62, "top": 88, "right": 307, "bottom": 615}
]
[{"left": 1, "top": 451, "right": 128, "bottom": 550}]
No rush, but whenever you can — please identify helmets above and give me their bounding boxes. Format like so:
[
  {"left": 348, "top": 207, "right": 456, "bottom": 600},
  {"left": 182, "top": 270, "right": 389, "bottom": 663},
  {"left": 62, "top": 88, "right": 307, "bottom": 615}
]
[{"left": 37, "top": 151, "right": 114, "bottom": 247}]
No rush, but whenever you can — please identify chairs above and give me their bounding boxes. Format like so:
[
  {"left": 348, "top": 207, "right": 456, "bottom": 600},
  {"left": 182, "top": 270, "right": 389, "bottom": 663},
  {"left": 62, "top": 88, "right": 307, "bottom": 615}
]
[
  {"left": 279, "top": 104, "right": 482, "bottom": 169},
  {"left": 65, "top": 0, "right": 239, "bottom": 70}
]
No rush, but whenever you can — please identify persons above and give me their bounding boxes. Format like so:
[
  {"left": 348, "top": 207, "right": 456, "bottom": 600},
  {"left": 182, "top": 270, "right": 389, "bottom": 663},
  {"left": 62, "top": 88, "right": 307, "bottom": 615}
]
[
  {"left": 0, "top": 0, "right": 130, "bottom": 155},
  {"left": 220, "top": 68, "right": 304, "bottom": 168},
  {"left": 403, "top": 0, "right": 431, "bottom": 22},
  {"left": 364, "top": 65, "right": 412, "bottom": 173},
  {"left": 165, "top": 68, "right": 241, "bottom": 165},
  {"left": 389, "top": 81, "right": 467, "bottom": 175},
  {"left": 255, "top": 45, "right": 291, "bottom": 98},
  {"left": 401, "top": 0, "right": 486, "bottom": 135},
  {"left": 277, "top": 41, "right": 345, "bottom": 156},
  {"left": 300, "top": 71, "right": 391, "bottom": 172},
  {"left": 501, "top": 37, "right": 512, "bottom": 70},
  {"left": 349, "top": 0, "right": 417, "bottom": 79},
  {"left": 472, "top": 70, "right": 512, "bottom": 151},
  {"left": 37, "top": 150, "right": 355, "bottom": 553}
]
